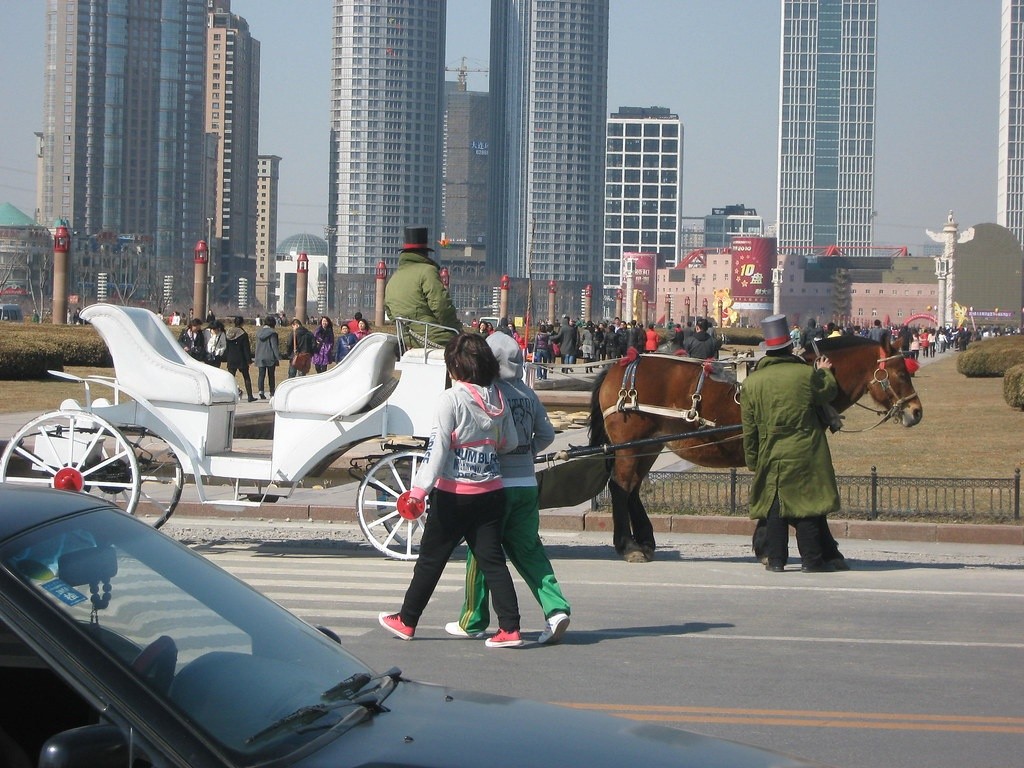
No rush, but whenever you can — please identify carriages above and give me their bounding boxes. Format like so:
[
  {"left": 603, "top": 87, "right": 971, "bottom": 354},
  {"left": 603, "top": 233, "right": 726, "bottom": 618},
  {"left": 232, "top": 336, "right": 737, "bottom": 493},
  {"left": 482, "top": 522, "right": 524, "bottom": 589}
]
[{"left": 0, "top": 300, "right": 925, "bottom": 562}]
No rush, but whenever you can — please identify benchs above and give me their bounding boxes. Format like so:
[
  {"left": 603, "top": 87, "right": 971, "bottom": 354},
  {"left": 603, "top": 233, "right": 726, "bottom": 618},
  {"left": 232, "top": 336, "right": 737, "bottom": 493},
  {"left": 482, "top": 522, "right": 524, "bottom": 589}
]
[
  {"left": 79, "top": 302, "right": 239, "bottom": 407},
  {"left": 394, "top": 317, "right": 459, "bottom": 363},
  {"left": 273, "top": 331, "right": 402, "bottom": 415}
]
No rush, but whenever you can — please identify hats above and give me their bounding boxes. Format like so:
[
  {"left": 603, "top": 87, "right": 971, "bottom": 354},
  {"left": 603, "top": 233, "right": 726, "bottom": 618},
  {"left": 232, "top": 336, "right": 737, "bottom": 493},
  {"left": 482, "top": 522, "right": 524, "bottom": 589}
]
[
  {"left": 759, "top": 314, "right": 800, "bottom": 351},
  {"left": 398, "top": 226, "right": 435, "bottom": 252}
]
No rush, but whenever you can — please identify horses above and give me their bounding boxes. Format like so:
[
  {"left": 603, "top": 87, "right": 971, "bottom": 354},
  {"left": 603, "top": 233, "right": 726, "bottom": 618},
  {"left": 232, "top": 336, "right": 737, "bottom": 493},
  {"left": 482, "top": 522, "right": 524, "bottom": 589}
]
[{"left": 593, "top": 334, "right": 925, "bottom": 564}]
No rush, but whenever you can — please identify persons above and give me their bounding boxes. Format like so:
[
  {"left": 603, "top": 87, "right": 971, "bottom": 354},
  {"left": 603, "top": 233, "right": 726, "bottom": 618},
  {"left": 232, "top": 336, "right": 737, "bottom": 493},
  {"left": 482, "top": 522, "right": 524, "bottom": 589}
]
[
  {"left": 536, "top": 316, "right": 660, "bottom": 380},
  {"left": 384, "top": 226, "right": 463, "bottom": 349},
  {"left": 207, "top": 310, "right": 215, "bottom": 322},
  {"left": 32, "top": 308, "right": 39, "bottom": 322},
  {"left": 248, "top": 315, "right": 263, "bottom": 326},
  {"left": 790, "top": 318, "right": 1016, "bottom": 361},
  {"left": 472, "top": 317, "right": 524, "bottom": 350},
  {"left": 157, "top": 308, "right": 193, "bottom": 326},
  {"left": 445, "top": 332, "right": 570, "bottom": 644},
  {"left": 67, "top": 308, "right": 81, "bottom": 324},
  {"left": 666, "top": 318, "right": 722, "bottom": 360},
  {"left": 740, "top": 315, "right": 842, "bottom": 573},
  {"left": 275, "top": 314, "right": 288, "bottom": 327},
  {"left": 255, "top": 316, "right": 280, "bottom": 399},
  {"left": 177, "top": 317, "right": 258, "bottom": 402},
  {"left": 286, "top": 313, "right": 370, "bottom": 378},
  {"left": 379, "top": 333, "right": 524, "bottom": 647}
]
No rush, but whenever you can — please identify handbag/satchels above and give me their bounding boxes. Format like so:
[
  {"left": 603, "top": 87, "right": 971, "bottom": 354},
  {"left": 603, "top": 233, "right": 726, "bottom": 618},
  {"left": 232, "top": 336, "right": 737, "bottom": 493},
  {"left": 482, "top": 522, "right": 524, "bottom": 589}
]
[
  {"left": 190, "top": 346, "right": 205, "bottom": 361},
  {"left": 204, "top": 352, "right": 216, "bottom": 362},
  {"left": 291, "top": 351, "right": 311, "bottom": 373}
]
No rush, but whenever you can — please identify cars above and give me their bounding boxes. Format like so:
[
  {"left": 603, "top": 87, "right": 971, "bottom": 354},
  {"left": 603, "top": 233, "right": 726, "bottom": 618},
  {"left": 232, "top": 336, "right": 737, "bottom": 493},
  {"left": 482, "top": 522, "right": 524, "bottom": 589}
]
[{"left": 1, "top": 479, "right": 813, "bottom": 768}]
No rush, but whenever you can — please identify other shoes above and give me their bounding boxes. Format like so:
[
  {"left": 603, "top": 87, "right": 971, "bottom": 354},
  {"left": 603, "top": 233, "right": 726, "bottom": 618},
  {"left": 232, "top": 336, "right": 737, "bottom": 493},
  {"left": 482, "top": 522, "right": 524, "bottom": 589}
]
[
  {"left": 248, "top": 396, "right": 258, "bottom": 402},
  {"left": 539, "top": 376, "right": 547, "bottom": 380},
  {"left": 586, "top": 370, "right": 594, "bottom": 373},
  {"left": 802, "top": 563, "right": 841, "bottom": 574},
  {"left": 765, "top": 558, "right": 785, "bottom": 572},
  {"left": 259, "top": 393, "right": 266, "bottom": 400},
  {"left": 570, "top": 367, "right": 574, "bottom": 372}
]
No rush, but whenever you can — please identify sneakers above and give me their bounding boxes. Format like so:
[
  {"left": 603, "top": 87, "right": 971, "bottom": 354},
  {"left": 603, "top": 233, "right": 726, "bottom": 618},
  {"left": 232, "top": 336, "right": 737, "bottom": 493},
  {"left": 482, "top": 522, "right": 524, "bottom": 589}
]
[
  {"left": 485, "top": 628, "right": 524, "bottom": 648},
  {"left": 445, "top": 621, "right": 487, "bottom": 636},
  {"left": 538, "top": 612, "right": 570, "bottom": 644},
  {"left": 379, "top": 612, "right": 414, "bottom": 641}
]
